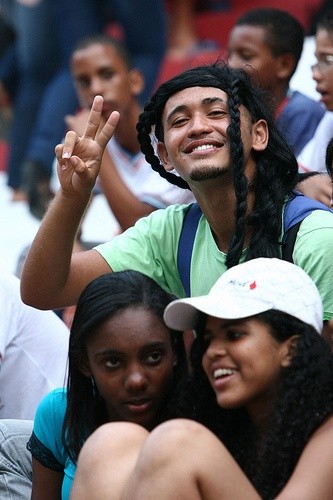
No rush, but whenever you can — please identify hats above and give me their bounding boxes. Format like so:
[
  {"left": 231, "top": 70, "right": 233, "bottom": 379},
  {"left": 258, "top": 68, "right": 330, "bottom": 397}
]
[{"left": 163, "top": 257, "right": 324, "bottom": 336}]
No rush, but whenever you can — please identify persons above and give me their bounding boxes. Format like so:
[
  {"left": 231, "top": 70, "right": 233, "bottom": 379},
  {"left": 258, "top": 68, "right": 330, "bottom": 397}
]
[
  {"left": 0, "top": 0, "right": 172, "bottom": 329},
  {"left": 156, "top": 0, "right": 333, "bottom": 207},
  {"left": 20, "top": 52, "right": 333, "bottom": 344},
  {"left": 26, "top": 270, "right": 193, "bottom": 500},
  {"left": 0, "top": 269, "right": 70, "bottom": 500},
  {"left": 71, "top": 257, "right": 333, "bottom": 500}
]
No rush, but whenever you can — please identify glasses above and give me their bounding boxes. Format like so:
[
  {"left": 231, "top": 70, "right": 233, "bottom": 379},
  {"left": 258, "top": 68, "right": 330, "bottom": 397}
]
[{"left": 311, "top": 54, "right": 332, "bottom": 69}]
